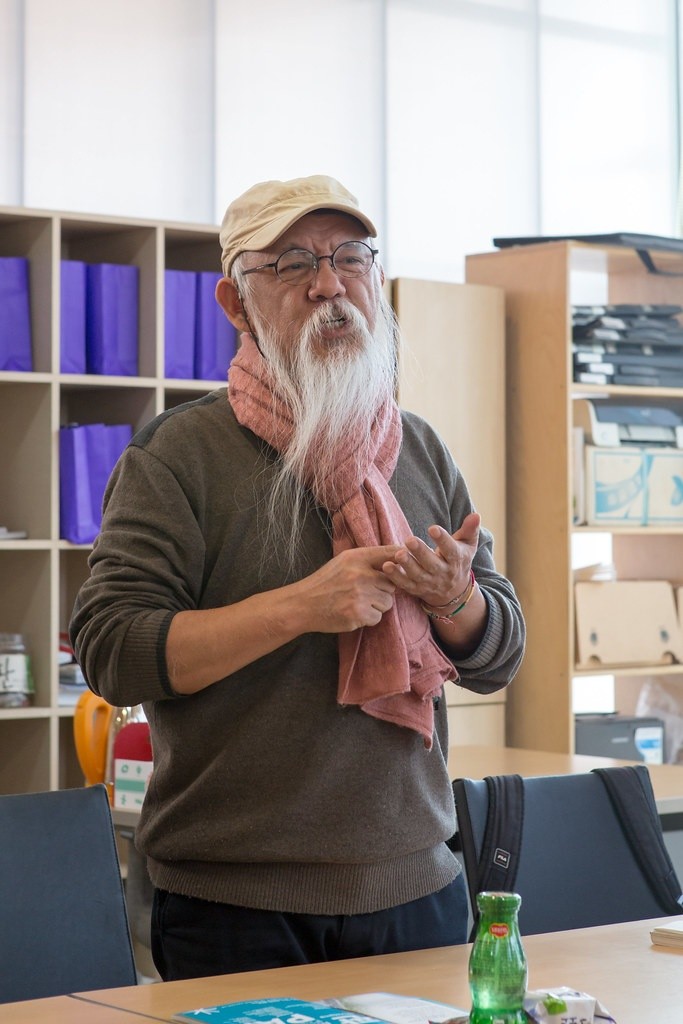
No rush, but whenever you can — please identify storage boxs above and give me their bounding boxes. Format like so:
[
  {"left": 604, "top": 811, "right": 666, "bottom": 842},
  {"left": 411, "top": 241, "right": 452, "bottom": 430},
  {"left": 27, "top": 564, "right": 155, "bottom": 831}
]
[
  {"left": 584, "top": 448, "right": 683, "bottom": 529},
  {"left": 574, "top": 718, "right": 665, "bottom": 764},
  {"left": 114, "top": 759, "right": 154, "bottom": 811}
]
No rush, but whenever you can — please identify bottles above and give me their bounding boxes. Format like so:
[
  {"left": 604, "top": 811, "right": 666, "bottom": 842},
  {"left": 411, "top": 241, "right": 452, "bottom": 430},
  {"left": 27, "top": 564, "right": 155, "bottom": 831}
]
[{"left": 469, "top": 892, "right": 527, "bottom": 1024}]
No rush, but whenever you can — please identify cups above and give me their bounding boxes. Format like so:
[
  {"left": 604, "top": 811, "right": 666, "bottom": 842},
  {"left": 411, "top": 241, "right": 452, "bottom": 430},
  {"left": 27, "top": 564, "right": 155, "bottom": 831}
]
[{"left": 0, "top": 633, "right": 33, "bottom": 710}]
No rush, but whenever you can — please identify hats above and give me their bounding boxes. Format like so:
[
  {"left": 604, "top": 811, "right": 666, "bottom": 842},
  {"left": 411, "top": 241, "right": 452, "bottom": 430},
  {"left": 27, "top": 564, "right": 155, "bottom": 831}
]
[{"left": 218, "top": 175, "right": 377, "bottom": 278}]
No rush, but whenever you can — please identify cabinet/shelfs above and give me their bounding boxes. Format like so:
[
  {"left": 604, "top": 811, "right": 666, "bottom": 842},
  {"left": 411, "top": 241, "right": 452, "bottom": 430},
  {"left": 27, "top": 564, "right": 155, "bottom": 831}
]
[
  {"left": 466, "top": 240, "right": 683, "bottom": 766},
  {"left": 0, "top": 205, "right": 507, "bottom": 796}
]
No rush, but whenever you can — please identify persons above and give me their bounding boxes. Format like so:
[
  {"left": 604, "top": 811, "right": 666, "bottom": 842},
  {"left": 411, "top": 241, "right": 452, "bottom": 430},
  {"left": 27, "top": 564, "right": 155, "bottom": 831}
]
[{"left": 67, "top": 169, "right": 527, "bottom": 993}]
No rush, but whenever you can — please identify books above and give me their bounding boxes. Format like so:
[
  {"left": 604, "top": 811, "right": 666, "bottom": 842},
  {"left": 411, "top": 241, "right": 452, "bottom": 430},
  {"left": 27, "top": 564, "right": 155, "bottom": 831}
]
[
  {"left": 59, "top": 630, "right": 88, "bottom": 687},
  {"left": 574, "top": 705, "right": 665, "bottom": 764},
  {"left": 568, "top": 299, "right": 683, "bottom": 527},
  {"left": 171, "top": 991, "right": 472, "bottom": 1024}
]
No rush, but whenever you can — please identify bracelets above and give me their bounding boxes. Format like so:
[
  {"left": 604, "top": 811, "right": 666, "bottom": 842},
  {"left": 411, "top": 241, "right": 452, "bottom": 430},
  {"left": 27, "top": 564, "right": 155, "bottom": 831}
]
[{"left": 419, "top": 566, "right": 475, "bottom": 631}]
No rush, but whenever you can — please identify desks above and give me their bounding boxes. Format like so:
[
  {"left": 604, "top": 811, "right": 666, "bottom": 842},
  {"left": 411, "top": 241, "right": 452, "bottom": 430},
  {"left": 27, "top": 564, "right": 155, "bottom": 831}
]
[
  {"left": 0, "top": 915, "right": 683, "bottom": 1023},
  {"left": 110, "top": 746, "right": 683, "bottom": 984}
]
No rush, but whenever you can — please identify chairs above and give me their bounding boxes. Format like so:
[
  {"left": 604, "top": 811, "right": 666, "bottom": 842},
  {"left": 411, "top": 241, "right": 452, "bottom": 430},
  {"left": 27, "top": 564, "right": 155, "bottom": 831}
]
[
  {"left": 453, "top": 765, "right": 683, "bottom": 936},
  {"left": 0, "top": 783, "right": 138, "bottom": 1005}
]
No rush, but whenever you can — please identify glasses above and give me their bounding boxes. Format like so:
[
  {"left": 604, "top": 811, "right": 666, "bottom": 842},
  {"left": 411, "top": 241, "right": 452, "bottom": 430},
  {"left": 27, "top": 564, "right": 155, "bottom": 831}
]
[{"left": 240, "top": 242, "right": 377, "bottom": 286}]
